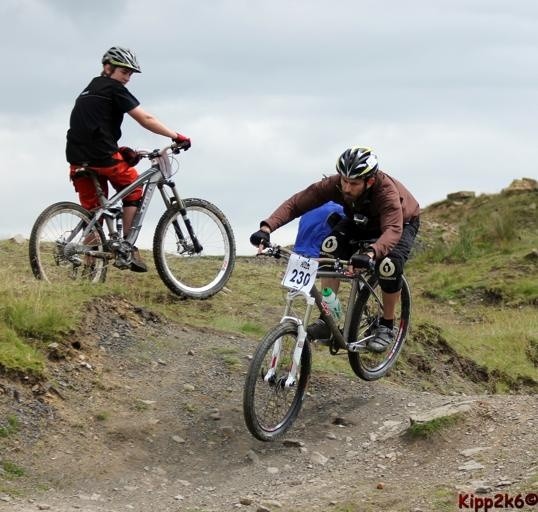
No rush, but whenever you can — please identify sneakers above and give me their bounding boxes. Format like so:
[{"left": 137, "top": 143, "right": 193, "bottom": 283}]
[
  {"left": 81, "top": 264, "right": 94, "bottom": 278},
  {"left": 367, "top": 320, "right": 395, "bottom": 352},
  {"left": 306, "top": 321, "right": 331, "bottom": 341},
  {"left": 129, "top": 258, "right": 148, "bottom": 273}
]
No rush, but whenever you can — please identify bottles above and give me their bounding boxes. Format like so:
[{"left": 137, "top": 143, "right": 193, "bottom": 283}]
[{"left": 319, "top": 286, "right": 344, "bottom": 327}]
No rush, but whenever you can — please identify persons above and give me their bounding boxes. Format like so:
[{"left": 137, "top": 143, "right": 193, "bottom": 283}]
[
  {"left": 250, "top": 146, "right": 421, "bottom": 353},
  {"left": 65, "top": 46, "right": 191, "bottom": 280}
]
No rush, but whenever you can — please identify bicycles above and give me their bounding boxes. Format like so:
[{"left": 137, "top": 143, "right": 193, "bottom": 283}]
[
  {"left": 29, "top": 138, "right": 236, "bottom": 300},
  {"left": 243, "top": 214, "right": 410, "bottom": 442}
]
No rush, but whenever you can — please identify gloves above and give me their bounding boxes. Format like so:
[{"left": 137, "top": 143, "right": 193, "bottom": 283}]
[
  {"left": 347, "top": 253, "right": 370, "bottom": 273},
  {"left": 250, "top": 230, "right": 270, "bottom": 250},
  {"left": 172, "top": 132, "right": 191, "bottom": 151}
]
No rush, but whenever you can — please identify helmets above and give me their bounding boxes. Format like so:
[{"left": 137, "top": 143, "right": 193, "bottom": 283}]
[
  {"left": 336, "top": 147, "right": 378, "bottom": 180},
  {"left": 102, "top": 47, "right": 142, "bottom": 74}
]
